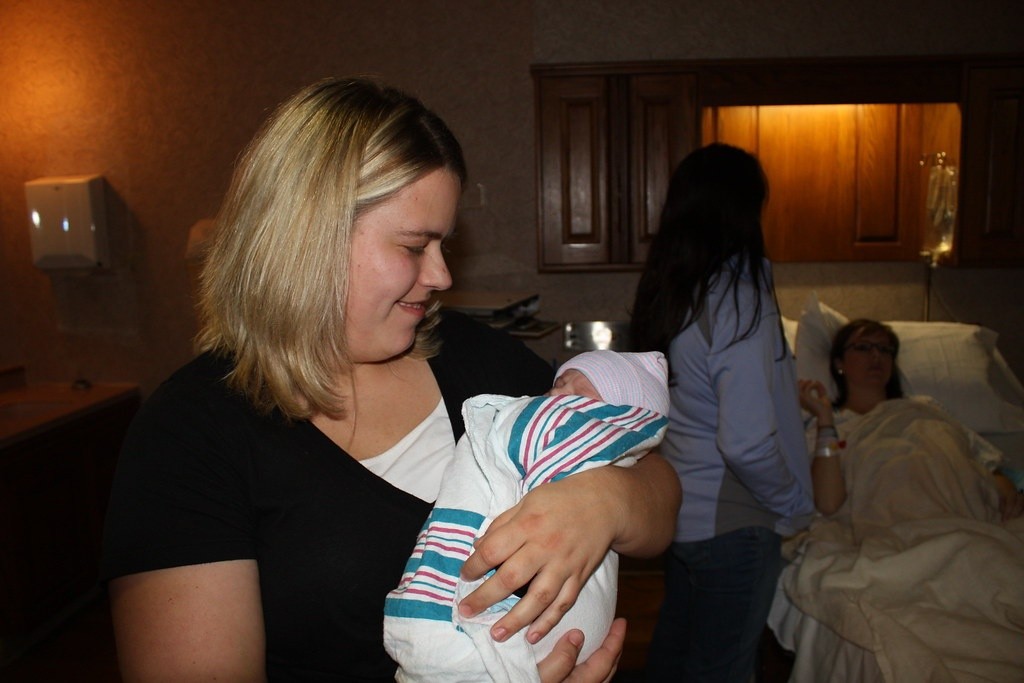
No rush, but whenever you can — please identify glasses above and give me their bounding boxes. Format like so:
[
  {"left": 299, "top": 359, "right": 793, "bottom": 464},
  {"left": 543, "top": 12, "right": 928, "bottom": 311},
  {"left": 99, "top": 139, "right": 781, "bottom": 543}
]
[{"left": 843, "top": 340, "right": 896, "bottom": 356}]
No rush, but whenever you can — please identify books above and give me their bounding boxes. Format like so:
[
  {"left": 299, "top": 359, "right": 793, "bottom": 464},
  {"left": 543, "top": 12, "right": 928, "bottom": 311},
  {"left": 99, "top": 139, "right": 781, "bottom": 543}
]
[{"left": 508, "top": 320, "right": 558, "bottom": 339}]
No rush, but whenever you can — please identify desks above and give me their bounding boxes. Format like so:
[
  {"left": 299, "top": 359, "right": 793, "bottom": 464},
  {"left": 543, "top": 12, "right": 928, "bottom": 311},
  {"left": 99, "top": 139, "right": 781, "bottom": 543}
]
[{"left": 420, "top": 286, "right": 562, "bottom": 340}]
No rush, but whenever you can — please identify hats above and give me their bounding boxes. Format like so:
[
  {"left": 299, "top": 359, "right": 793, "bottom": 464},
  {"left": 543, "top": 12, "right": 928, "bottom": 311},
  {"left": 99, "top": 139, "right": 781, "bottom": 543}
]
[{"left": 553, "top": 349, "right": 670, "bottom": 419}]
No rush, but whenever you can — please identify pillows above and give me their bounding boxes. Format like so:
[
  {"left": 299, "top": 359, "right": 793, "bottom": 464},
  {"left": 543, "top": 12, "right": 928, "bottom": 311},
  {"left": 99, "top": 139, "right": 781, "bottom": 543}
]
[{"left": 790, "top": 288, "right": 1024, "bottom": 434}]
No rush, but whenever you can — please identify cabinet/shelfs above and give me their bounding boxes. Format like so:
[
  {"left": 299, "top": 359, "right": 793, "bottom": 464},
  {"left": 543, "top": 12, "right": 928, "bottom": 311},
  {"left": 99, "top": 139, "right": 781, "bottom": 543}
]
[{"left": 529, "top": 51, "right": 1024, "bottom": 271}]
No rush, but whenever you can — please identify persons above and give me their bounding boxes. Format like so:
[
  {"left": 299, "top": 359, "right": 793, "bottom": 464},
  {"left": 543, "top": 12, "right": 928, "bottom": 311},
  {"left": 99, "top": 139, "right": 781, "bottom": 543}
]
[
  {"left": 383, "top": 350, "right": 670, "bottom": 683},
  {"left": 624, "top": 141, "right": 815, "bottom": 683},
  {"left": 100, "top": 73, "right": 682, "bottom": 683},
  {"left": 799, "top": 318, "right": 1024, "bottom": 522}
]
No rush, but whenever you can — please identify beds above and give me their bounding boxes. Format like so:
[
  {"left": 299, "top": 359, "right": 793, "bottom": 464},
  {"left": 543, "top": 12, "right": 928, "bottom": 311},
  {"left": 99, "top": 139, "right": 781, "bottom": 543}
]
[{"left": 766, "top": 319, "right": 1024, "bottom": 682}]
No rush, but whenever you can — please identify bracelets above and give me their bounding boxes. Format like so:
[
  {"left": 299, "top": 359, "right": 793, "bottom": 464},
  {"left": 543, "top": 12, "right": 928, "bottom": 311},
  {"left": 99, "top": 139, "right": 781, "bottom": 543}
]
[{"left": 816, "top": 427, "right": 845, "bottom": 458}]
